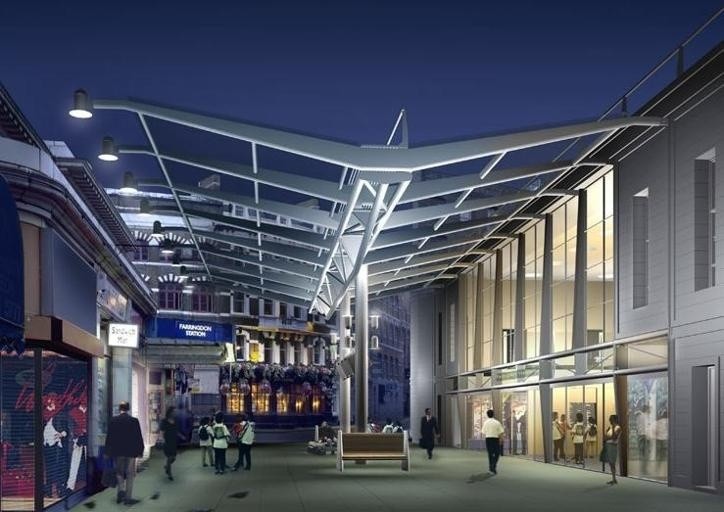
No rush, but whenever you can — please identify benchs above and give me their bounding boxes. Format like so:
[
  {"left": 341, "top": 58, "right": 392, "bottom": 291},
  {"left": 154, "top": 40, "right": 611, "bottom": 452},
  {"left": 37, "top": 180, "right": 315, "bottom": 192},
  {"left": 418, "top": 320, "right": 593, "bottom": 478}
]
[{"left": 314, "top": 424, "right": 410, "bottom": 472}]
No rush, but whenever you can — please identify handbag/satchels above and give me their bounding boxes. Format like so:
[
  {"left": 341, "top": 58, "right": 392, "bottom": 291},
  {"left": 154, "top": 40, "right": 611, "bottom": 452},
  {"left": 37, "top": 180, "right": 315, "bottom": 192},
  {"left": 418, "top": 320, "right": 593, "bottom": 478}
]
[
  {"left": 419, "top": 438, "right": 426, "bottom": 450},
  {"left": 101, "top": 465, "right": 115, "bottom": 485},
  {"left": 598, "top": 449, "right": 609, "bottom": 462}
]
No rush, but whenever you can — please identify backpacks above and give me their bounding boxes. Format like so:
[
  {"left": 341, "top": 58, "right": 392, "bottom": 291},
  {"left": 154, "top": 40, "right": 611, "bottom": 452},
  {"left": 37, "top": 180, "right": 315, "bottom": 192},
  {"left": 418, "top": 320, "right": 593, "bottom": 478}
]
[
  {"left": 575, "top": 425, "right": 584, "bottom": 435},
  {"left": 588, "top": 426, "right": 597, "bottom": 437},
  {"left": 232, "top": 423, "right": 244, "bottom": 441},
  {"left": 214, "top": 426, "right": 224, "bottom": 440},
  {"left": 387, "top": 426, "right": 393, "bottom": 434},
  {"left": 199, "top": 426, "right": 209, "bottom": 441},
  {"left": 396, "top": 427, "right": 403, "bottom": 433}
]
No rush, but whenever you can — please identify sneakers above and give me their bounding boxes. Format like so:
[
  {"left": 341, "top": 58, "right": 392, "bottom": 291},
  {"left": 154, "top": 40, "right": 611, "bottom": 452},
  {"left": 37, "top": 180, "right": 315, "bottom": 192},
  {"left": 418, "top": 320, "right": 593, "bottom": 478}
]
[
  {"left": 164, "top": 466, "right": 174, "bottom": 482},
  {"left": 428, "top": 454, "right": 432, "bottom": 459},
  {"left": 604, "top": 481, "right": 617, "bottom": 485},
  {"left": 202, "top": 463, "right": 250, "bottom": 474},
  {"left": 117, "top": 490, "right": 140, "bottom": 506},
  {"left": 488, "top": 469, "right": 497, "bottom": 475},
  {"left": 554, "top": 456, "right": 596, "bottom": 464}
]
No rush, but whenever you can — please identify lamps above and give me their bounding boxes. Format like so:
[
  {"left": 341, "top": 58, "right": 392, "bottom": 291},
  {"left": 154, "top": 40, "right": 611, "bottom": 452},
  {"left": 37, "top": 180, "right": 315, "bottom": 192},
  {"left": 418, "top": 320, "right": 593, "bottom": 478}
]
[
  {"left": 68, "top": 88, "right": 174, "bottom": 254},
  {"left": 341, "top": 314, "right": 378, "bottom": 349}
]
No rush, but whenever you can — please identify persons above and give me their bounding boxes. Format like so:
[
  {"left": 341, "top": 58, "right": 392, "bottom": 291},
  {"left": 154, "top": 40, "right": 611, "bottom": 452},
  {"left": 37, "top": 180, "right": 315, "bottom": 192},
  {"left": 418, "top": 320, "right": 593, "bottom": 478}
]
[
  {"left": 102, "top": 400, "right": 146, "bottom": 505},
  {"left": 635, "top": 404, "right": 651, "bottom": 474},
  {"left": 197, "top": 411, "right": 254, "bottom": 475},
  {"left": 367, "top": 417, "right": 404, "bottom": 433},
  {"left": 480, "top": 409, "right": 505, "bottom": 475},
  {"left": 504, "top": 408, "right": 596, "bottom": 464},
  {"left": 420, "top": 406, "right": 439, "bottom": 460},
  {"left": 159, "top": 403, "right": 186, "bottom": 480},
  {"left": 604, "top": 414, "right": 621, "bottom": 483},
  {"left": 648, "top": 411, "right": 669, "bottom": 473}
]
